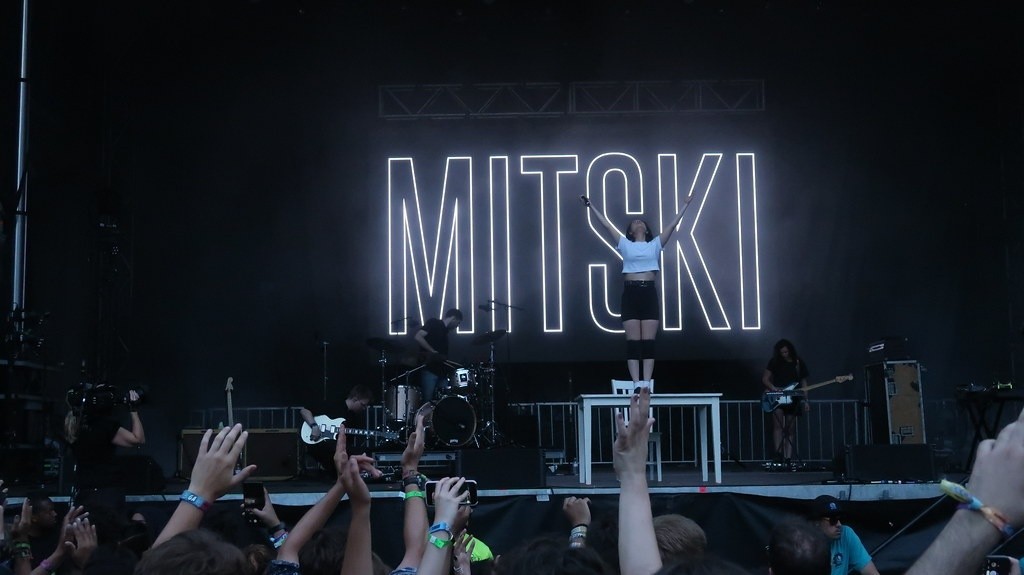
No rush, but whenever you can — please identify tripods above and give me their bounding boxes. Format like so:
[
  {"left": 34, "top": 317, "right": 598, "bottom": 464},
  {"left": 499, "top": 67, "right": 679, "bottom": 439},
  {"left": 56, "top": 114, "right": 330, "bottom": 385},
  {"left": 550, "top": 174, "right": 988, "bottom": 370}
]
[
  {"left": 360, "top": 349, "right": 406, "bottom": 448},
  {"left": 468, "top": 341, "right": 525, "bottom": 448}
]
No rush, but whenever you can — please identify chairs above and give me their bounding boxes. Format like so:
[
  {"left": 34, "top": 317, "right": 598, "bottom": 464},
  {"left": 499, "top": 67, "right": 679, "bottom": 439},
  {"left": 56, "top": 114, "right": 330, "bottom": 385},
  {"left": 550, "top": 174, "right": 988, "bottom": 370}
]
[{"left": 610, "top": 379, "right": 663, "bottom": 482}]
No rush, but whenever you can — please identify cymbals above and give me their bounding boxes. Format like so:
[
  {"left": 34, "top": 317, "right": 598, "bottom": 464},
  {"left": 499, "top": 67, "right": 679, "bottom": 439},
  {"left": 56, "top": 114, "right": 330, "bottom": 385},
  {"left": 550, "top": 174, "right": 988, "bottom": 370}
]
[
  {"left": 366, "top": 336, "right": 405, "bottom": 351},
  {"left": 472, "top": 327, "right": 507, "bottom": 346}
]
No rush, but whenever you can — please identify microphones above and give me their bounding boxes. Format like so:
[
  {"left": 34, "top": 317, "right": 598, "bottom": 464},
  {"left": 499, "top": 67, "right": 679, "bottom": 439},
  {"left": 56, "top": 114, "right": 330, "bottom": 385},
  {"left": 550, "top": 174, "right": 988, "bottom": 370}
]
[
  {"left": 580, "top": 195, "right": 590, "bottom": 206},
  {"left": 478, "top": 304, "right": 493, "bottom": 312}
]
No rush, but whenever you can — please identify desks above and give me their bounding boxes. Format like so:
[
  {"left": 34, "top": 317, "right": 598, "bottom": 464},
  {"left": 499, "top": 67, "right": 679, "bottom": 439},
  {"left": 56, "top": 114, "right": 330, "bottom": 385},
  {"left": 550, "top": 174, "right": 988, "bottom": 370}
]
[{"left": 574, "top": 392, "right": 724, "bottom": 486}]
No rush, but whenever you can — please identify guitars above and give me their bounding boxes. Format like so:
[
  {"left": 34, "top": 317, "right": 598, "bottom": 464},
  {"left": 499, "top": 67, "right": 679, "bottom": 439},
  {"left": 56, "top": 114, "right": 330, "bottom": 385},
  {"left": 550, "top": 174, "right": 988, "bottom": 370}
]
[
  {"left": 301, "top": 415, "right": 402, "bottom": 445},
  {"left": 760, "top": 373, "right": 854, "bottom": 412}
]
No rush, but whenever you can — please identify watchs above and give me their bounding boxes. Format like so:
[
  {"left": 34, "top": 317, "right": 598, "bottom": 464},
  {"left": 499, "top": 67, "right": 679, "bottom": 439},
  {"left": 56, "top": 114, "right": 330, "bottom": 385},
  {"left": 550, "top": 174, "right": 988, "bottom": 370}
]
[
  {"left": 267, "top": 522, "right": 288, "bottom": 534},
  {"left": 402, "top": 473, "right": 426, "bottom": 490}
]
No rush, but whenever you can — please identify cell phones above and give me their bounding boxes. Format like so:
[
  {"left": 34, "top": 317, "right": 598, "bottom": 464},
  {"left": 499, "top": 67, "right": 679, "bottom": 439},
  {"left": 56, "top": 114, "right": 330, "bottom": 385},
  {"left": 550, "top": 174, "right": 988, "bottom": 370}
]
[
  {"left": 244, "top": 482, "right": 265, "bottom": 528},
  {"left": 425, "top": 480, "right": 478, "bottom": 507}
]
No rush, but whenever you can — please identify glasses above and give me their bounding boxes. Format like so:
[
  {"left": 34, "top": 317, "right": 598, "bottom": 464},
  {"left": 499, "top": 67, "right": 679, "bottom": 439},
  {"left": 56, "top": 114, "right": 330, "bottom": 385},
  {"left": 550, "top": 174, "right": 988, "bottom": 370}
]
[
  {"left": 359, "top": 400, "right": 367, "bottom": 407},
  {"left": 822, "top": 517, "right": 842, "bottom": 526}
]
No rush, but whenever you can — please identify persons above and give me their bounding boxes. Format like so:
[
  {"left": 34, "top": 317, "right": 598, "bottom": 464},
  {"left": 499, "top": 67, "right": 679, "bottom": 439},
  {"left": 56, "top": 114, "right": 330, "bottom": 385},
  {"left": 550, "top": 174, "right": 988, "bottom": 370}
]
[
  {"left": 762, "top": 339, "right": 810, "bottom": 472},
  {"left": 414, "top": 308, "right": 463, "bottom": 399},
  {"left": 287, "top": 385, "right": 373, "bottom": 482},
  {"left": 575, "top": 189, "right": 697, "bottom": 394},
  {"left": 0, "top": 383, "right": 1024, "bottom": 575}
]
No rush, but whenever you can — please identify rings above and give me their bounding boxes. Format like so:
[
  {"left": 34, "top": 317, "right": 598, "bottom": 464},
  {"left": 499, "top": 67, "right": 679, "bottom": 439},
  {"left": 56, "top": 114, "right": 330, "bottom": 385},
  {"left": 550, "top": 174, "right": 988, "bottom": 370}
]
[{"left": 453, "top": 565, "right": 463, "bottom": 575}]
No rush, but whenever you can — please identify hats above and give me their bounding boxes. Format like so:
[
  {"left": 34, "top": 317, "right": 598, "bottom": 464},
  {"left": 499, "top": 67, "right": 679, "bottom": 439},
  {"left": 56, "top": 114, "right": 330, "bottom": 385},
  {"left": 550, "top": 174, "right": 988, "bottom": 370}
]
[{"left": 811, "top": 495, "right": 842, "bottom": 514}]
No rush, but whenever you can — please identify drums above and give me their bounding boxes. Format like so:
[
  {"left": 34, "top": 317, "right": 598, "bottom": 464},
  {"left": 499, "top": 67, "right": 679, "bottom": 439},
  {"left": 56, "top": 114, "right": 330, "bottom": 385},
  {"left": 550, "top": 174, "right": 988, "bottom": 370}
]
[
  {"left": 382, "top": 385, "right": 422, "bottom": 429},
  {"left": 415, "top": 393, "right": 478, "bottom": 447},
  {"left": 439, "top": 364, "right": 477, "bottom": 394}
]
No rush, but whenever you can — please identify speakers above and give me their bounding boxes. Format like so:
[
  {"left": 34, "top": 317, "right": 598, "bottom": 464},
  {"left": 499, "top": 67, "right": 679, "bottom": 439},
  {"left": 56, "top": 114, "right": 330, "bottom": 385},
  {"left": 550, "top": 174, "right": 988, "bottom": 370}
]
[
  {"left": 242, "top": 429, "right": 301, "bottom": 480},
  {"left": 846, "top": 443, "right": 943, "bottom": 484},
  {"left": 864, "top": 359, "right": 926, "bottom": 444},
  {"left": 461, "top": 446, "right": 548, "bottom": 490},
  {"left": 178, "top": 428, "right": 225, "bottom": 480}
]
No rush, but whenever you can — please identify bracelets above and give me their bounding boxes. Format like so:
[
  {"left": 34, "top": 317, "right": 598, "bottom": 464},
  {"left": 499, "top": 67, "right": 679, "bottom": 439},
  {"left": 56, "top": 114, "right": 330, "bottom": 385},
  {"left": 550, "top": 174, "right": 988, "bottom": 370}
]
[
  {"left": 568, "top": 523, "right": 588, "bottom": 548},
  {"left": 805, "top": 399, "right": 808, "bottom": 402},
  {"left": 309, "top": 423, "right": 317, "bottom": 428},
  {"left": 179, "top": 489, "right": 212, "bottom": 512},
  {"left": 428, "top": 522, "right": 453, "bottom": 536},
  {"left": 403, "top": 491, "right": 426, "bottom": 501},
  {"left": 940, "top": 478, "right": 1015, "bottom": 540},
  {"left": 269, "top": 532, "right": 289, "bottom": 549},
  {"left": 40, "top": 560, "right": 56, "bottom": 575},
  {"left": 427, "top": 534, "right": 453, "bottom": 548},
  {"left": 10, "top": 541, "right": 33, "bottom": 559}
]
